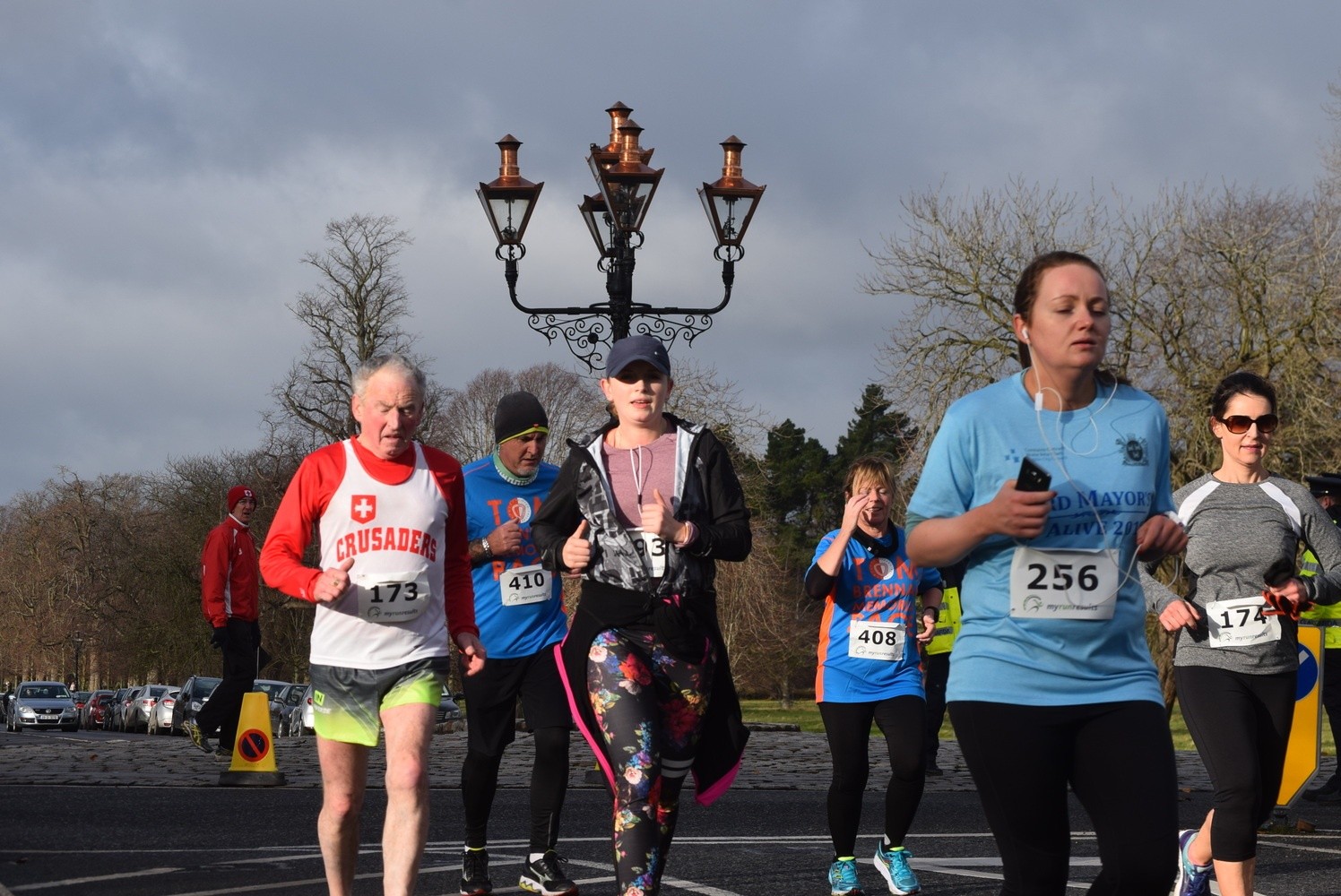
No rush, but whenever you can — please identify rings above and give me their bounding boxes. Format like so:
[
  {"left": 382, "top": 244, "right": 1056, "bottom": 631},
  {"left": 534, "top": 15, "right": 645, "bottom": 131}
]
[{"left": 333, "top": 580, "right": 340, "bottom": 586}]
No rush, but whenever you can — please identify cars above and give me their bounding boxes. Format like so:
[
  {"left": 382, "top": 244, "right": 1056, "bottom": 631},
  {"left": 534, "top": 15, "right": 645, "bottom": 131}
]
[{"left": 0, "top": 675, "right": 465, "bottom": 739}]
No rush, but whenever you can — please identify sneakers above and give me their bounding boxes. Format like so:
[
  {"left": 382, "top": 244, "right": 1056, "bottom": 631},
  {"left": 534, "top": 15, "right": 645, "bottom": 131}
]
[
  {"left": 873, "top": 840, "right": 921, "bottom": 895},
  {"left": 181, "top": 716, "right": 213, "bottom": 753},
  {"left": 828, "top": 854, "right": 865, "bottom": 896},
  {"left": 215, "top": 745, "right": 232, "bottom": 758},
  {"left": 519, "top": 850, "right": 579, "bottom": 896},
  {"left": 1172, "top": 828, "right": 1211, "bottom": 896},
  {"left": 460, "top": 848, "right": 493, "bottom": 895}
]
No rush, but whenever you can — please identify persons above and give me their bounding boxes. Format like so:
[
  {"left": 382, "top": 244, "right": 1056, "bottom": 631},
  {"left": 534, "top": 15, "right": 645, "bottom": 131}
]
[
  {"left": 802, "top": 454, "right": 944, "bottom": 896},
  {"left": 901, "top": 251, "right": 1191, "bottom": 896},
  {"left": 1294, "top": 475, "right": 1341, "bottom": 796},
  {"left": 264, "top": 351, "right": 488, "bottom": 894},
  {"left": 532, "top": 333, "right": 754, "bottom": 896},
  {"left": 920, "top": 577, "right": 966, "bottom": 775},
  {"left": 451, "top": 389, "right": 583, "bottom": 896},
  {"left": 182, "top": 483, "right": 260, "bottom": 762},
  {"left": 1132, "top": 373, "right": 1341, "bottom": 895}
]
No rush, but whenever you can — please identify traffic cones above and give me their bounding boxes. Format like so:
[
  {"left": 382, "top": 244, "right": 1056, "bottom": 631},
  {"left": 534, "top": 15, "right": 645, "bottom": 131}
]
[{"left": 218, "top": 691, "right": 286, "bottom": 786}]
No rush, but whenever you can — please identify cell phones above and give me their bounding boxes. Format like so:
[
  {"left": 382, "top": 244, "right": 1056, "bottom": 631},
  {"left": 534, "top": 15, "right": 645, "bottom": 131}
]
[{"left": 1015, "top": 456, "right": 1054, "bottom": 492}]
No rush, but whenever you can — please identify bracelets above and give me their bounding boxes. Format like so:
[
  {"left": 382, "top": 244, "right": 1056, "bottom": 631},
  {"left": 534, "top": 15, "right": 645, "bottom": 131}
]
[{"left": 482, "top": 536, "right": 492, "bottom": 560}]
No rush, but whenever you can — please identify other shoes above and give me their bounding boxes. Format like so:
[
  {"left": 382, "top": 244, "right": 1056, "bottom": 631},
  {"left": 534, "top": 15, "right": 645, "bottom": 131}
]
[
  {"left": 1319, "top": 789, "right": 1341, "bottom": 805},
  {"left": 1302, "top": 775, "right": 1339, "bottom": 801}
]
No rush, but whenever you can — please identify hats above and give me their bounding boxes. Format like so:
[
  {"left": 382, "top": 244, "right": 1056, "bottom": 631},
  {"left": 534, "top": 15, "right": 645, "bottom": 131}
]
[
  {"left": 228, "top": 486, "right": 258, "bottom": 514},
  {"left": 493, "top": 390, "right": 549, "bottom": 444},
  {"left": 605, "top": 336, "right": 670, "bottom": 377},
  {"left": 1303, "top": 475, "right": 1340, "bottom": 499}
]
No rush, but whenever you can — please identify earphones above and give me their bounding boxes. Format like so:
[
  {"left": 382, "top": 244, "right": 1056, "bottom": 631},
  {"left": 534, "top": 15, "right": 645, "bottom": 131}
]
[{"left": 1021, "top": 326, "right": 1029, "bottom": 340}]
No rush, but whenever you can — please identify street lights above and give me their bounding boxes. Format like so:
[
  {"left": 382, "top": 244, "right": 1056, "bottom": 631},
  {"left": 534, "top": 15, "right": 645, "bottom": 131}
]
[{"left": 477, "top": 99, "right": 766, "bottom": 371}]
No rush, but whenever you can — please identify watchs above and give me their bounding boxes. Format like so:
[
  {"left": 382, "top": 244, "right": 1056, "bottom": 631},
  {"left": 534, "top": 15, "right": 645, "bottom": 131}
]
[{"left": 925, "top": 606, "right": 940, "bottom": 623}]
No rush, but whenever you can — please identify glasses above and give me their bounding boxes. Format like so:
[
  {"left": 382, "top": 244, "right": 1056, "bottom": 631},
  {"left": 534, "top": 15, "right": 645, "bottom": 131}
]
[{"left": 1218, "top": 414, "right": 1279, "bottom": 434}]
